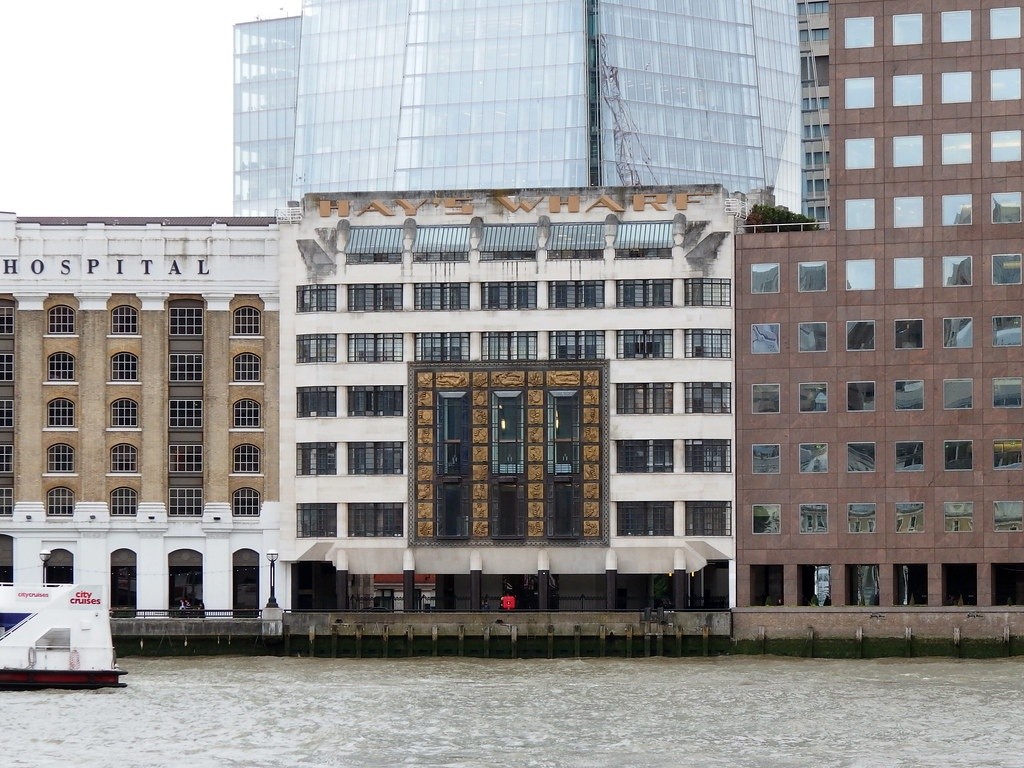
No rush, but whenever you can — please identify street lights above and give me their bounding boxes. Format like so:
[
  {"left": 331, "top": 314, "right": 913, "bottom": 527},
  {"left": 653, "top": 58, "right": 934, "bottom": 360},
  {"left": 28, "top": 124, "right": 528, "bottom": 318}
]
[
  {"left": 39, "top": 550, "right": 53, "bottom": 588},
  {"left": 266, "top": 550, "right": 282, "bottom": 610}
]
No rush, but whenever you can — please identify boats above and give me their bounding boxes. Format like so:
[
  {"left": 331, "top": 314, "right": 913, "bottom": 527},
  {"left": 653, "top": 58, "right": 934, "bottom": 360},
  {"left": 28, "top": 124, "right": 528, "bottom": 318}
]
[{"left": 0, "top": 580, "right": 130, "bottom": 691}]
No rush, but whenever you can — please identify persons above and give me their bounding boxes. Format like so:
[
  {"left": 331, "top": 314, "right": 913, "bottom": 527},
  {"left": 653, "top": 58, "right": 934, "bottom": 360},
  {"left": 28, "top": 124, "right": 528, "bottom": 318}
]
[{"left": 177, "top": 598, "right": 205, "bottom": 619}]
